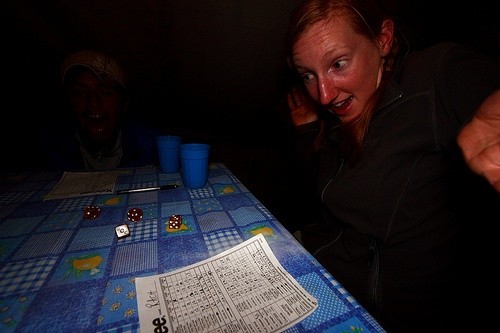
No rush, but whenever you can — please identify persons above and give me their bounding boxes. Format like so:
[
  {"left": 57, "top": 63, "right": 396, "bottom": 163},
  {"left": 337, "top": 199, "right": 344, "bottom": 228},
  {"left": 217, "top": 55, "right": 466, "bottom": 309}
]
[
  {"left": 267, "top": 0, "right": 500, "bottom": 327},
  {"left": 57, "top": 47, "right": 158, "bottom": 172}
]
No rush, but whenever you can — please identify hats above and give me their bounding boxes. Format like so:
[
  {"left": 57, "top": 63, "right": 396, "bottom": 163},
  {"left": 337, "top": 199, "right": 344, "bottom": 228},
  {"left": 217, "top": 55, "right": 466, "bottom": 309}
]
[{"left": 59, "top": 50, "right": 126, "bottom": 89}]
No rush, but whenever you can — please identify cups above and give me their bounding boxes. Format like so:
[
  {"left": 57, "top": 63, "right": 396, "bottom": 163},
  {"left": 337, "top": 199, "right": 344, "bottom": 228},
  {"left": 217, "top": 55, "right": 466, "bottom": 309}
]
[
  {"left": 157, "top": 136, "right": 181, "bottom": 172},
  {"left": 180, "top": 143, "right": 210, "bottom": 189}
]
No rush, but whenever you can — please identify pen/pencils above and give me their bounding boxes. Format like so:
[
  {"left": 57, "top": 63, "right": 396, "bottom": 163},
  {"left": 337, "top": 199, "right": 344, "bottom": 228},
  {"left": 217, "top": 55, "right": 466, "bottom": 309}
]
[{"left": 113, "top": 184, "right": 179, "bottom": 194}]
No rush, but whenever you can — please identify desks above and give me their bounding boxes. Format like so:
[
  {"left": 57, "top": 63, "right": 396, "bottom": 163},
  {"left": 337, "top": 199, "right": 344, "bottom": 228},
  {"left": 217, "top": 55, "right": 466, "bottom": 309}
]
[{"left": 0, "top": 163, "right": 386, "bottom": 333}]
